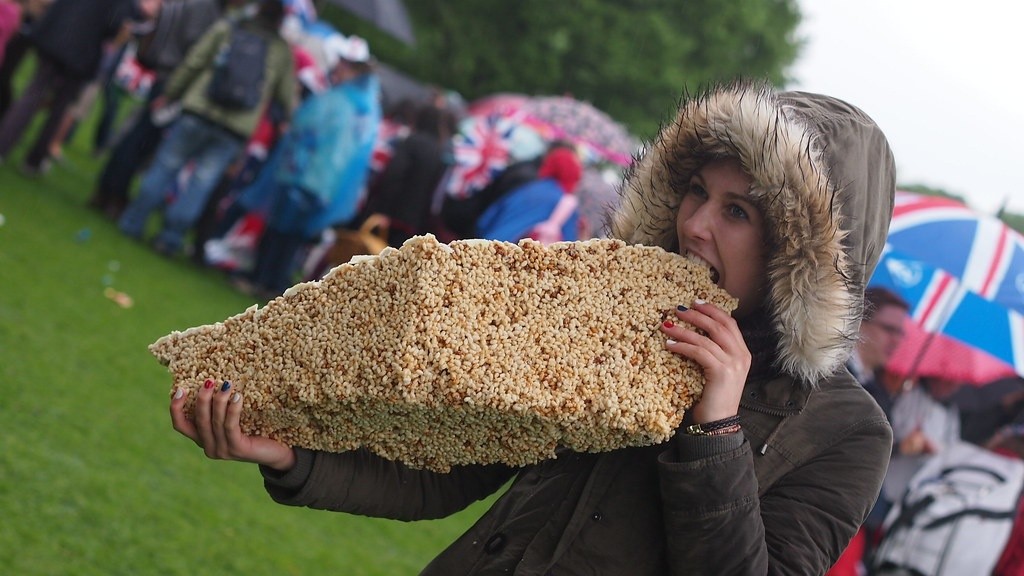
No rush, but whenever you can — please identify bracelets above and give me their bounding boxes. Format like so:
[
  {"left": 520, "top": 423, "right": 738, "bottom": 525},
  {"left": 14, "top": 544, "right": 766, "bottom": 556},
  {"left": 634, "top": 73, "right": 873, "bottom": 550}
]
[{"left": 684, "top": 414, "right": 741, "bottom": 435}]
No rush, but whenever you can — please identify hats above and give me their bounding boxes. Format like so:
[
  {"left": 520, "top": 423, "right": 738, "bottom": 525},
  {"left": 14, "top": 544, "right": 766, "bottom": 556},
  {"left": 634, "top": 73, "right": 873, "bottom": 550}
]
[{"left": 330, "top": 29, "right": 371, "bottom": 63}]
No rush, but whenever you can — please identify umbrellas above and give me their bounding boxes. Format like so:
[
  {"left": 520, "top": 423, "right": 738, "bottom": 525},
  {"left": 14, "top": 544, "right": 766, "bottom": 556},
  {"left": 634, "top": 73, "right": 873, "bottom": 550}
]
[
  {"left": 447, "top": 77, "right": 647, "bottom": 231},
  {"left": 334, "top": 0, "right": 418, "bottom": 50},
  {"left": 865, "top": 189, "right": 1022, "bottom": 382}
]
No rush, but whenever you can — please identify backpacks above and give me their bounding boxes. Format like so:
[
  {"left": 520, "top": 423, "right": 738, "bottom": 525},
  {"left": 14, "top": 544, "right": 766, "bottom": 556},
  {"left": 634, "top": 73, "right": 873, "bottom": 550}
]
[{"left": 210, "top": 18, "right": 275, "bottom": 111}]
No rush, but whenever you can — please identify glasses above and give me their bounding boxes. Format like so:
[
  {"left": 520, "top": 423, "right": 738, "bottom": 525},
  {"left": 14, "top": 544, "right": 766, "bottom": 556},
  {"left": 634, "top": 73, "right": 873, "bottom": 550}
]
[{"left": 872, "top": 318, "right": 906, "bottom": 339}]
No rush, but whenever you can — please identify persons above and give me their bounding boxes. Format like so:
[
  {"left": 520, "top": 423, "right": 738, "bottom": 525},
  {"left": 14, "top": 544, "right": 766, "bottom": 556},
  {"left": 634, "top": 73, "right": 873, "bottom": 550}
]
[
  {"left": 0, "top": 0, "right": 583, "bottom": 292},
  {"left": 826, "top": 287, "right": 1024, "bottom": 576},
  {"left": 167, "top": 65, "right": 895, "bottom": 576}
]
